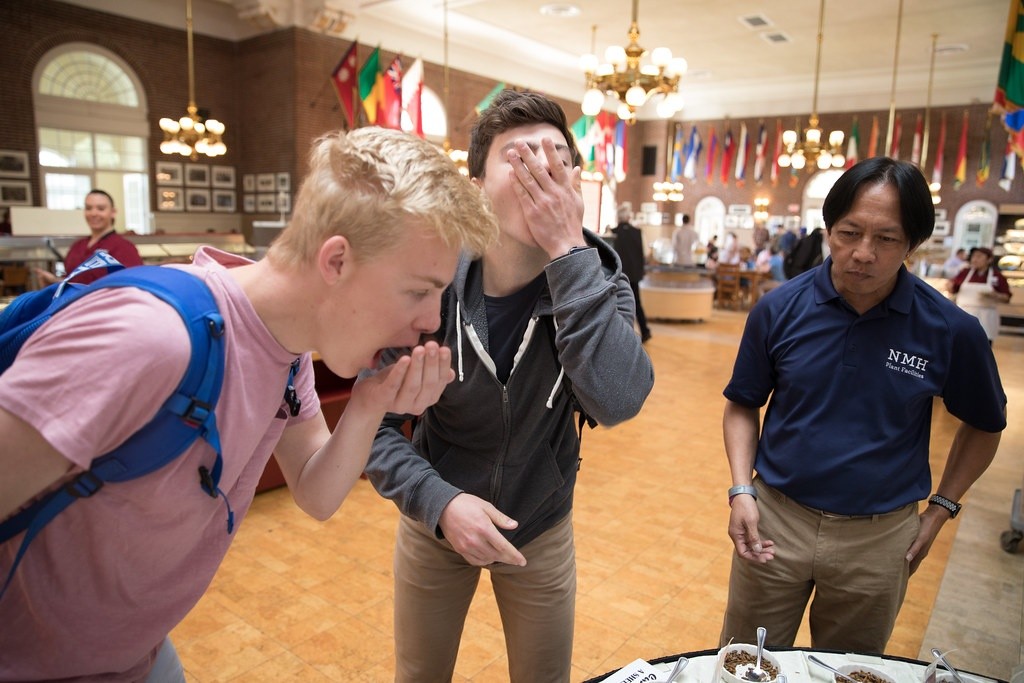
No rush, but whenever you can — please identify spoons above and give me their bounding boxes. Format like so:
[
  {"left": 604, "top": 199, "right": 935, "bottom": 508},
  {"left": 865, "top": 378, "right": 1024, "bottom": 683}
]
[{"left": 748, "top": 627, "right": 767, "bottom": 682}]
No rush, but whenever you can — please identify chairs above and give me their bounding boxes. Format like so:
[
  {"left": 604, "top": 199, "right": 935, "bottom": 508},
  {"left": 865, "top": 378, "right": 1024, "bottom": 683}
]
[{"left": 716, "top": 262, "right": 743, "bottom": 310}]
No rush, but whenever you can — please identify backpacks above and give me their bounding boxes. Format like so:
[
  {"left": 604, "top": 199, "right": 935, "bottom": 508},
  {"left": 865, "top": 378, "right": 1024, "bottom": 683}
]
[
  {"left": 784, "top": 228, "right": 824, "bottom": 279},
  {"left": 0, "top": 247, "right": 302, "bottom": 600}
]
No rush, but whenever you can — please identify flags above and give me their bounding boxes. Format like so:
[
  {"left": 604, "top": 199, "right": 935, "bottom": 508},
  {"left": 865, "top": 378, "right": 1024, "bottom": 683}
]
[
  {"left": 330, "top": 39, "right": 361, "bottom": 130},
  {"left": 567, "top": 109, "right": 630, "bottom": 182},
  {"left": 992, "top": 0, "right": 1024, "bottom": 168},
  {"left": 402, "top": 54, "right": 423, "bottom": 138},
  {"left": 668, "top": 109, "right": 1015, "bottom": 190},
  {"left": 357, "top": 45, "right": 384, "bottom": 124},
  {"left": 382, "top": 53, "right": 402, "bottom": 131},
  {"left": 475, "top": 83, "right": 504, "bottom": 115}
]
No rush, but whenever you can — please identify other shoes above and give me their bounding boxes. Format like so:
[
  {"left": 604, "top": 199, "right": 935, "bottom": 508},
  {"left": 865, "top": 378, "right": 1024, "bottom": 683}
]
[{"left": 641, "top": 330, "right": 651, "bottom": 343}]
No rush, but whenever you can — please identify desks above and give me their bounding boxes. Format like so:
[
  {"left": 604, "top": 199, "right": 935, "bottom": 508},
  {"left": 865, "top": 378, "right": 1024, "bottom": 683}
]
[
  {"left": 580, "top": 644, "right": 1007, "bottom": 683},
  {"left": 716, "top": 269, "right": 772, "bottom": 309}
]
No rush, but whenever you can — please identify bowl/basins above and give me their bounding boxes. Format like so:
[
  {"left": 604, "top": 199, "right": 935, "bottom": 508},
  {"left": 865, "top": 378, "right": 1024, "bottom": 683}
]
[
  {"left": 833, "top": 664, "right": 896, "bottom": 683},
  {"left": 719, "top": 644, "right": 781, "bottom": 683},
  {"left": 936, "top": 673, "right": 983, "bottom": 683}
]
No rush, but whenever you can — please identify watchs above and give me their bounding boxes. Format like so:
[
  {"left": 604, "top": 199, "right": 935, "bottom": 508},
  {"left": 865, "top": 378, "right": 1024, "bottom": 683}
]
[
  {"left": 550, "top": 246, "right": 590, "bottom": 263},
  {"left": 928, "top": 494, "right": 962, "bottom": 519}
]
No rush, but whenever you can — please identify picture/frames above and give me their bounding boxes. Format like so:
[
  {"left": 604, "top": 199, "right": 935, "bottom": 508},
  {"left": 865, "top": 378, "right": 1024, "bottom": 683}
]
[
  {"left": 0, "top": 181, "right": 35, "bottom": 206},
  {"left": 155, "top": 160, "right": 291, "bottom": 214},
  {"left": 0, "top": 150, "right": 30, "bottom": 179}
]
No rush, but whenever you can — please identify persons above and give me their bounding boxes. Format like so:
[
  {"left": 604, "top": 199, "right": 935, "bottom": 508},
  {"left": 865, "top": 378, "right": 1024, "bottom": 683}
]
[
  {"left": 612, "top": 208, "right": 651, "bottom": 344},
  {"left": 671, "top": 213, "right": 701, "bottom": 269},
  {"left": 28, "top": 190, "right": 143, "bottom": 288},
  {"left": 354, "top": 88, "right": 655, "bottom": 683},
  {"left": 0, "top": 126, "right": 499, "bottom": 682},
  {"left": 947, "top": 247, "right": 1012, "bottom": 349},
  {"left": 603, "top": 225, "right": 616, "bottom": 238},
  {"left": 942, "top": 247, "right": 977, "bottom": 280},
  {"left": 706, "top": 219, "right": 831, "bottom": 308},
  {"left": 716, "top": 156, "right": 1007, "bottom": 656}
]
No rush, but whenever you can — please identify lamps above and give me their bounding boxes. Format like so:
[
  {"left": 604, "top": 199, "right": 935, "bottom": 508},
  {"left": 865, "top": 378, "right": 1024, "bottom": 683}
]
[
  {"left": 156, "top": 0, "right": 229, "bottom": 160},
  {"left": 579, "top": 0, "right": 692, "bottom": 124},
  {"left": 777, "top": 0, "right": 848, "bottom": 172},
  {"left": 439, "top": 0, "right": 469, "bottom": 177},
  {"left": 920, "top": 32, "right": 942, "bottom": 205}
]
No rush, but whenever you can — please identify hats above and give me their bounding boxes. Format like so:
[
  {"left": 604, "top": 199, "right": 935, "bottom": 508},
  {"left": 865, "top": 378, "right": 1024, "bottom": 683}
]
[{"left": 970, "top": 247, "right": 993, "bottom": 258}]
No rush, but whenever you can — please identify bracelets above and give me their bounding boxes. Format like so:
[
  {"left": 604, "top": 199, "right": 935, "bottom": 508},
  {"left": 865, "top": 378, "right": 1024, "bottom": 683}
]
[{"left": 728, "top": 485, "right": 758, "bottom": 507}]
